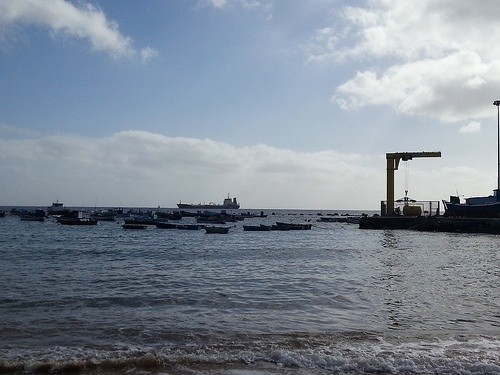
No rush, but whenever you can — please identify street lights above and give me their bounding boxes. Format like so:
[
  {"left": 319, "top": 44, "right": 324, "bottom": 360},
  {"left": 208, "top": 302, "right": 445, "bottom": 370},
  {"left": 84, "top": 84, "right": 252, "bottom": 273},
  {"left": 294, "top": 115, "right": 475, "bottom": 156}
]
[{"left": 493, "top": 100, "right": 500, "bottom": 191}]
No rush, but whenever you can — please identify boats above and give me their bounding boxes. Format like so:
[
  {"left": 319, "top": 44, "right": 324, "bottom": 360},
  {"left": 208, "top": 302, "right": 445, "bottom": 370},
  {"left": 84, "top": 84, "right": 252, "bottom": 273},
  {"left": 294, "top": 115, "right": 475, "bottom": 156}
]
[
  {"left": 176, "top": 192, "right": 241, "bottom": 209},
  {"left": 0, "top": 200, "right": 313, "bottom": 234},
  {"left": 442, "top": 199, "right": 500, "bottom": 218}
]
